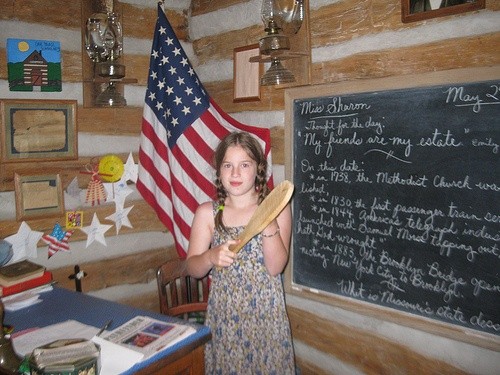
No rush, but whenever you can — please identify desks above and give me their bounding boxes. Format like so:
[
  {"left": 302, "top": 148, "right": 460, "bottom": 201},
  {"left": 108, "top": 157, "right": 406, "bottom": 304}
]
[{"left": 0, "top": 286, "right": 213, "bottom": 375}]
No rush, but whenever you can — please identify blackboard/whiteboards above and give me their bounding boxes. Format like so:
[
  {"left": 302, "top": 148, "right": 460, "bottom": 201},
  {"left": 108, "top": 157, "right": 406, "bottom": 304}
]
[{"left": 283, "top": 67, "right": 500, "bottom": 350}]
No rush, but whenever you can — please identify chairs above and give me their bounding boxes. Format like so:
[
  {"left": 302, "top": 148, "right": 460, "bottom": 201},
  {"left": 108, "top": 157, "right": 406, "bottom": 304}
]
[{"left": 157, "top": 258, "right": 209, "bottom": 316}]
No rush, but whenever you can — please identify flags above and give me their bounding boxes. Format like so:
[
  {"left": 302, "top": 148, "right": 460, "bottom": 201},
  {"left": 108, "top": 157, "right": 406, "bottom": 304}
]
[{"left": 136, "top": 0, "right": 274, "bottom": 306}]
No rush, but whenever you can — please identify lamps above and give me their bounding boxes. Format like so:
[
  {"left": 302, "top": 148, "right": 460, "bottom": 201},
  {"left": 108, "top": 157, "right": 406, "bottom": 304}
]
[
  {"left": 80, "top": 0, "right": 137, "bottom": 108},
  {"left": 254, "top": 0, "right": 313, "bottom": 89}
]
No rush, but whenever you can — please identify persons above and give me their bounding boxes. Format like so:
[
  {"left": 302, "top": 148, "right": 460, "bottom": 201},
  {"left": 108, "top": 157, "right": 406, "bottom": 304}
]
[{"left": 183, "top": 132, "right": 297, "bottom": 375}]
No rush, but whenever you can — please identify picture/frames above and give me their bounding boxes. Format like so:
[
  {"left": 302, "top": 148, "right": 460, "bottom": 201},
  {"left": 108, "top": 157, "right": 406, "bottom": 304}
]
[
  {"left": 401, "top": 0, "right": 485, "bottom": 24},
  {"left": 14, "top": 167, "right": 65, "bottom": 222},
  {"left": 0, "top": 98, "right": 78, "bottom": 163},
  {"left": 233, "top": 44, "right": 265, "bottom": 103}
]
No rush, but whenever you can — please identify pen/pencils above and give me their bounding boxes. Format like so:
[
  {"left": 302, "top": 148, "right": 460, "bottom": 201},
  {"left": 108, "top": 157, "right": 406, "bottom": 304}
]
[{"left": 96, "top": 320, "right": 112, "bottom": 336}]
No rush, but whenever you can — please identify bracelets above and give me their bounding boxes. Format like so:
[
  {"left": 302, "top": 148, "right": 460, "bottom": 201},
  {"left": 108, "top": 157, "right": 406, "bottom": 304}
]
[{"left": 262, "top": 226, "right": 280, "bottom": 237}]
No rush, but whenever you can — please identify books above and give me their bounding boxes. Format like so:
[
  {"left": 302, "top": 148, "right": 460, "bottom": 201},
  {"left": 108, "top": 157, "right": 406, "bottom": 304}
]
[
  {"left": 12, "top": 318, "right": 112, "bottom": 362},
  {"left": 91, "top": 314, "right": 197, "bottom": 375},
  {"left": 0, "top": 260, "right": 54, "bottom": 305}
]
